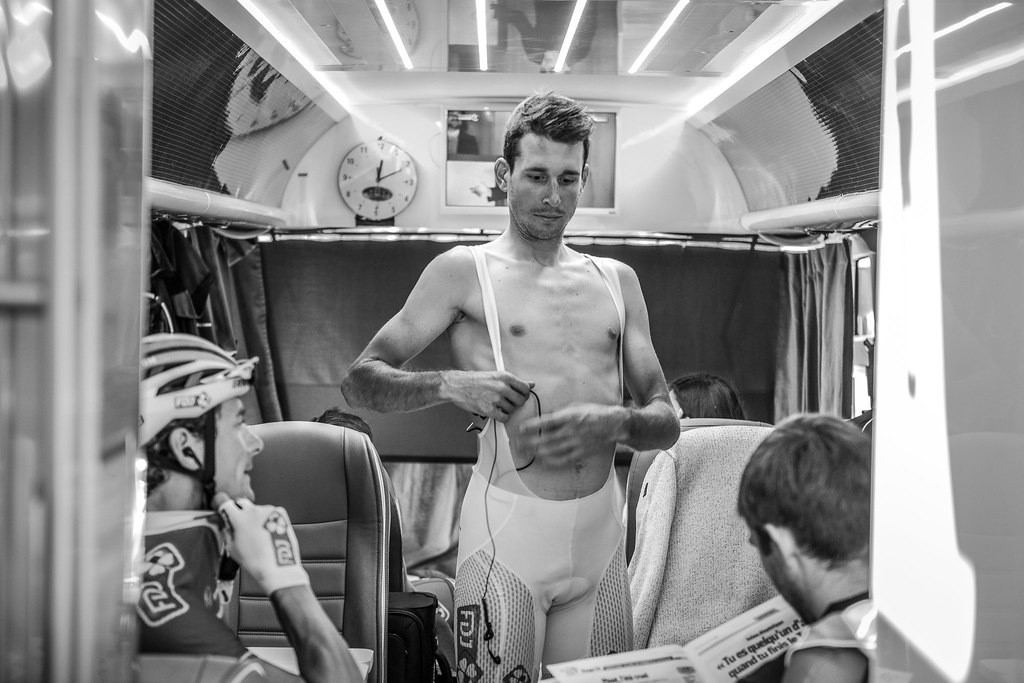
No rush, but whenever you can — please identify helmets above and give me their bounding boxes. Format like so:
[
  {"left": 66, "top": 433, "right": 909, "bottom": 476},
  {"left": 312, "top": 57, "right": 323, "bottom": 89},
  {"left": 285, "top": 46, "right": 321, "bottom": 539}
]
[{"left": 138, "top": 331, "right": 259, "bottom": 458}]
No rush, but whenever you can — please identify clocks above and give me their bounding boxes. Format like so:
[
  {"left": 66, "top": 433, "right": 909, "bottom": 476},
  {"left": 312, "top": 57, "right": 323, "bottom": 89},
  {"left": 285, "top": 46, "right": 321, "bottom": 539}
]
[
  {"left": 225, "top": 55, "right": 313, "bottom": 136},
  {"left": 337, "top": 141, "right": 417, "bottom": 221}
]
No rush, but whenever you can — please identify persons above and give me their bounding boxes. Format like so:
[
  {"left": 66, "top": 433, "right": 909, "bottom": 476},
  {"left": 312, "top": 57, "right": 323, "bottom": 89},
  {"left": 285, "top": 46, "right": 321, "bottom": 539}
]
[
  {"left": 338, "top": 91, "right": 680, "bottom": 683},
  {"left": 130, "top": 333, "right": 363, "bottom": 682},
  {"left": 312, "top": 408, "right": 374, "bottom": 442},
  {"left": 668, "top": 372, "right": 748, "bottom": 422},
  {"left": 735, "top": 413, "right": 876, "bottom": 683}
]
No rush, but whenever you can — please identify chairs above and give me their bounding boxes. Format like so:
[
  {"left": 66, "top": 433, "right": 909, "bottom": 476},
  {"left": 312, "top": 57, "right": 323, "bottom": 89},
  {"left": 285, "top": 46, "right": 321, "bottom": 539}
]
[
  {"left": 221, "top": 420, "right": 409, "bottom": 683},
  {"left": 627, "top": 417, "right": 784, "bottom": 650}
]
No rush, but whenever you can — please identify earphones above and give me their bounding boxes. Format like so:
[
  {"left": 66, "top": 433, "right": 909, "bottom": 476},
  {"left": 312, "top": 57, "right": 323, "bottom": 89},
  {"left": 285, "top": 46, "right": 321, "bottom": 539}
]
[
  {"left": 483, "top": 622, "right": 495, "bottom": 640},
  {"left": 488, "top": 650, "right": 501, "bottom": 664},
  {"left": 182, "top": 447, "right": 202, "bottom": 466}
]
